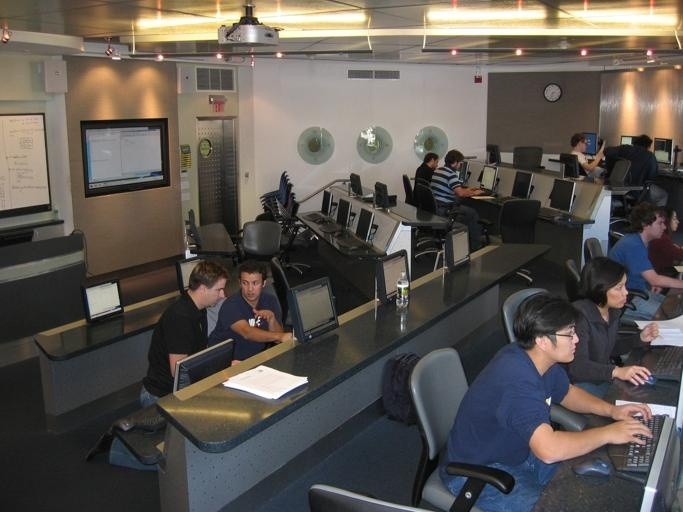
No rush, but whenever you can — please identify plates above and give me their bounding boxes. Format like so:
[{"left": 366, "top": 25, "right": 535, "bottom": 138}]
[
  {"left": 198, "top": 138, "right": 212, "bottom": 159},
  {"left": 356, "top": 126, "right": 394, "bottom": 165},
  {"left": 297, "top": 126, "right": 335, "bottom": 167},
  {"left": 412, "top": 125, "right": 448, "bottom": 164}
]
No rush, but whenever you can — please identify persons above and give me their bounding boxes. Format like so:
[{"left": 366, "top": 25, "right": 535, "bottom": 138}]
[
  {"left": 605, "top": 201, "right": 683, "bottom": 320},
  {"left": 648, "top": 207, "right": 683, "bottom": 274},
  {"left": 439, "top": 291, "right": 655, "bottom": 512},
  {"left": 602, "top": 135, "right": 669, "bottom": 209},
  {"left": 86, "top": 259, "right": 228, "bottom": 471},
  {"left": 208, "top": 261, "right": 298, "bottom": 366},
  {"left": 557, "top": 257, "right": 661, "bottom": 399},
  {"left": 414, "top": 152, "right": 439, "bottom": 195},
  {"left": 428, "top": 149, "right": 487, "bottom": 251},
  {"left": 570, "top": 132, "right": 606, "bottom": 176}
]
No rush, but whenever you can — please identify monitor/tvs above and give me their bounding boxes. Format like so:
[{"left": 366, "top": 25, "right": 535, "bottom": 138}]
[
  {"left": 376, "top": 250, "right": 410, "bottom": 303},
  {"left": 81, "top": 278, "right": 124, "bottom": 322},
  {"left": 172, "top": 339, "right": 236, "bottom": 392},
  {"left": 445, "top": 226, "right": 470, "bottom": 270},
  {"left": 176, "top": 255, "right": 208, "bottom": 296},
  {"left": 639, "top": 417, "right": 683, "bottom": 512},
  {"left": 286, "top": 277, "right": 340, "bottom": 342},
  {"left": 80, "top": 118, "right": 170, "bottom": 198}
]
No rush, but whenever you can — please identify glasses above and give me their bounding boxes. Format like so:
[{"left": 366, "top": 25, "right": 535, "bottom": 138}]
[
  {"left": 545, "top": 331, "right": 576, "bottom": 341},
  {"left": 582, "top": 142, "right": 587, "bottom": 146}
]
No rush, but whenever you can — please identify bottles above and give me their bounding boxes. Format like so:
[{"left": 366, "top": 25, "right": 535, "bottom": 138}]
[{"left": 394, "top": 272, "right": 409, "bottom": 311}]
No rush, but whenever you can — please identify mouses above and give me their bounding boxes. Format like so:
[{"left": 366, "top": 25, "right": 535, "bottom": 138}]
[
  {"left": 572, "top": 457, "right": 615, "bottom": 479},
  {"left": 348, "top": 246, "right": 360, "bottom": 251},
  {"left": 114, "top": 418, "right": 135, "bottom": 431},
  {"left": 636, "top": 375, "right": 657, "bottom": 386}
]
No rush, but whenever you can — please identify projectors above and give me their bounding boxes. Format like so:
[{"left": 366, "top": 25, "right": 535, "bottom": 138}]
[{"left": 218, "top": 25, "right": 279, "bottom": 46}]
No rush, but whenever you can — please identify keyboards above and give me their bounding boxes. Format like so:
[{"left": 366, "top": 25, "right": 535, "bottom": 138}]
[
  {"left": 134, "top": 403, "right": 167, "bottom": 432},
  {"left": 640, "top": 346, "right": 683, "bottom": 382},
  {"left": 608, "top": 414, "right": 669, "bottom": 474},
  {"left": 302, "top": 213, "right": 364, "bottom": 248}
]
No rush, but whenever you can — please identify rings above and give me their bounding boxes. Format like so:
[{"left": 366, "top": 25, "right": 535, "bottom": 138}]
[{"left": 631, "top": 374, "right": 636, "bottom": 378}]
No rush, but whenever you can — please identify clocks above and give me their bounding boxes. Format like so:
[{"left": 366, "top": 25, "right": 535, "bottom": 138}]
[{"left": 543, "top": 82, "right": 562, "bottom": 103}]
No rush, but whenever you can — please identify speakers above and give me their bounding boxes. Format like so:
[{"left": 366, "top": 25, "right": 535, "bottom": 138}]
[{"left": 43, "top": 60, "right": 68, "bottom": 94}]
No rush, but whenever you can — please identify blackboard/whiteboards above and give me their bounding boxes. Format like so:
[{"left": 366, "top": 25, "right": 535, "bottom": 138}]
[{"left": 0, "top": 112, "right": 51, "bottom": 211}]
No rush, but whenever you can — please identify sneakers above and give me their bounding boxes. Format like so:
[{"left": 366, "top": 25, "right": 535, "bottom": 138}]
[{"left": 83, "top": 431, "right": 113, "bottom": 463}]
